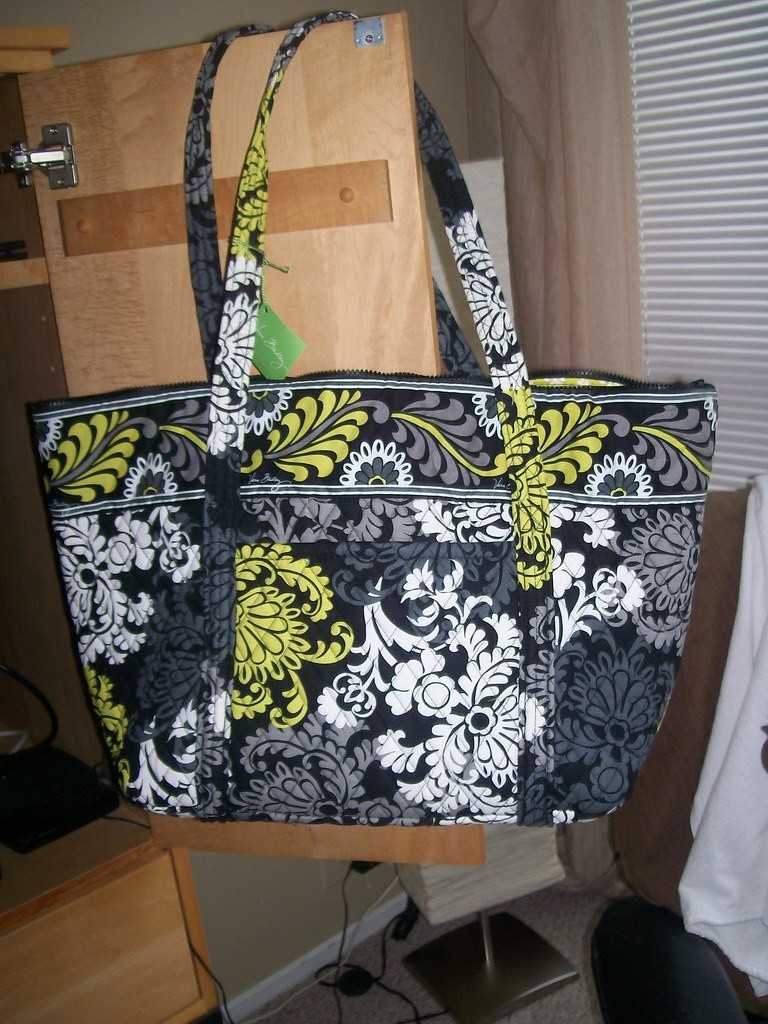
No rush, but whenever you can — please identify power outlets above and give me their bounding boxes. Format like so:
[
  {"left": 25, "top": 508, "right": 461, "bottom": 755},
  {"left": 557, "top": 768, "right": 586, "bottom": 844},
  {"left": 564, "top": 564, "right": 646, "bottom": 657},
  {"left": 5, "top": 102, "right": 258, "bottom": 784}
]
[{"left": 318, "top": 859, "right": 354, "bottom": 892}]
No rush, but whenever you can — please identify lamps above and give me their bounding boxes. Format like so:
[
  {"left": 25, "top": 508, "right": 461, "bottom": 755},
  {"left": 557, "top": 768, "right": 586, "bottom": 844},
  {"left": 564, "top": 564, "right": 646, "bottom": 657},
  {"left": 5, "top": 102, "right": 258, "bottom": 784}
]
[{"left": 394, "top": 822, "right": 581, "bottom": 1024}]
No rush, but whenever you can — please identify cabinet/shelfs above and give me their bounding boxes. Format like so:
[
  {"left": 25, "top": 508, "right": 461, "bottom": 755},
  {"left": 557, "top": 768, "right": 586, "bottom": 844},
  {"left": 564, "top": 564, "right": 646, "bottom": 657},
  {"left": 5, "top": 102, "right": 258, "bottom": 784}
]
[{"left": 1, "top": 11, "right": 489, "bottom": 1024}]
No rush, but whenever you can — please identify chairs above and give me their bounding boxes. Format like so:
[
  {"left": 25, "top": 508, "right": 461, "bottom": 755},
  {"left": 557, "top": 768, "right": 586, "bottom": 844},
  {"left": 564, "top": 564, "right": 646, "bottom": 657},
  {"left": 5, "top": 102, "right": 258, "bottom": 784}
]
[{"left": 591, "top": 483, "right": 768, "bottom": 1024}]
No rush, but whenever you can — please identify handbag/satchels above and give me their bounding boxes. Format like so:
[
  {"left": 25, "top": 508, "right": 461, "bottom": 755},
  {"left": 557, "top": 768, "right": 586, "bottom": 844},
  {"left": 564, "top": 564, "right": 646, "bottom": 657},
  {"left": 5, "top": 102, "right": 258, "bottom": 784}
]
[{"left": 30, "top": 8, "right": 719, "bottom": 826}]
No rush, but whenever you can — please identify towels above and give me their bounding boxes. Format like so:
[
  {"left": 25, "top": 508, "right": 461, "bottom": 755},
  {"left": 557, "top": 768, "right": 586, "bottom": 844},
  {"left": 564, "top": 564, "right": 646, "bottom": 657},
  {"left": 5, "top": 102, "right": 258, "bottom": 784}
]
[{"left": 678, "top": 473, "right": 767, "bottom": 1000}]
